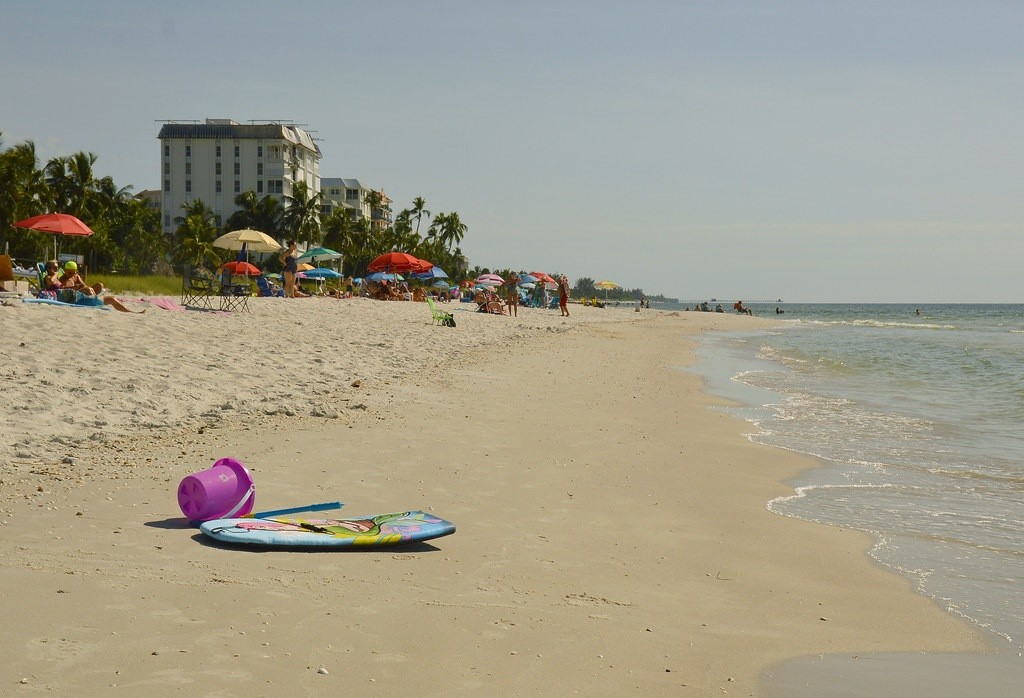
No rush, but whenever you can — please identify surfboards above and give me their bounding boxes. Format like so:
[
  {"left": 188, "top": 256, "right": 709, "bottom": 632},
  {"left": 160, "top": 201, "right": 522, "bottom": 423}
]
[{"left": 199, "top": 510, "right": 456, "bottom": 547}]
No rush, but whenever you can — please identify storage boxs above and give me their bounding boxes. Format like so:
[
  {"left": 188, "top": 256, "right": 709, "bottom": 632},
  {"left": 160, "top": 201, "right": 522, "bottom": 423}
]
[{"left": 462, "top": 298, "right": 470, "bottom": 302}]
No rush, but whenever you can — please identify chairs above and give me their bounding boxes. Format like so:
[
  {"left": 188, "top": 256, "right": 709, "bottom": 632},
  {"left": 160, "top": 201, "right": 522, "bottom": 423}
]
[
  {"left": 0, "top": 254, "right": 104, "bottom": 307},
  {"left": 734, "top": 303, "right": 746, "bottom": 315},
  {"left": 520, "top": 293, "right": 560, "bottom": 309},
  {"left": 255, "top": 275, "right": 285, "bottom": 298},
  {"left": 474, "top": 293, "right": 506, "bottom": 316},
  {"left": 427, "top": 297, "right": 452, "bottom": 326},
  {"left": 360, "top": 279, "right": 438, "bottom": 301},
  {"left": 701, "top": 303, "right": 708, "bottom": 311},
  {"left": 182, "top": 264, "right": 253, "bottom": 313}
]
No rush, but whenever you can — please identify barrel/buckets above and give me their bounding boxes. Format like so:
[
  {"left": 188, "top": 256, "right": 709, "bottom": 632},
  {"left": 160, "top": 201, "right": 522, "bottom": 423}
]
[{"left": 177, "top": 458, "right": 256, "bottom": 521}]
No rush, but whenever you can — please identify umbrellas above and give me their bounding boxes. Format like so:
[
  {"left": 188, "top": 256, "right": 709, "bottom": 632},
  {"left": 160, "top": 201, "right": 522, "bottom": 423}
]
[
  {"left": 594, "top": 279, "right": 619, "bottom": 300},
  {"left": 365, "top": 252, "right": 449, "bottom": 287},
  {"left": 213, "top": 226, "right": 283, "bottom": 285},
  {"left": 11, "top": 211, "right": 95, "bottom": 260},
  {"left": 297, "top": 246, "right": 344, "bottom": 296},
  {"left": 460, "top": 272, "right": 560, "bottom": 293}
]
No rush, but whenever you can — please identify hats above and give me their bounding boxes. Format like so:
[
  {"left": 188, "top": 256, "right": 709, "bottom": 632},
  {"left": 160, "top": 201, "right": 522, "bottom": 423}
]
[
  {"left": 387, "top": 279, "right": 393, "bottom": 283},
  {"left": 64, "top": 261, "right": 78, "bottom": 270}
]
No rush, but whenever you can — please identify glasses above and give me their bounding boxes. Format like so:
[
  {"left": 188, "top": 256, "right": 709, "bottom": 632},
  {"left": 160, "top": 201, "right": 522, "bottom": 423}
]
[
  {"left": 53, "top": 266, "right": 59, "bottom": 269},
  {"left": 67, "top": 272, "right": 76, "bottom": 274}
]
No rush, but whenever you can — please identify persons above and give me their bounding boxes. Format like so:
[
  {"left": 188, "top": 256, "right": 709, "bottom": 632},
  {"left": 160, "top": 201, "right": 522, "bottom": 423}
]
[
  {"left": 496, "top": 270, "right": 523, "bottom": 318},
  {"left": 557, "top": 274, "right": 570, "bottom": 317},
  {"left": 373, "top": 280, "right": 410, "bottom": 301},
  {"left": 916, "top": 308, "right": 920, "bottom": 315},
  {"left": 346, "top": 276, "right": 354, "bottom": 298},
  {"left": 640, "top": 297, "right": 650, "bottom": 309},
  {"left": 693, "top": 301, "right": 729, "bottom": 312},
  {"left": 279, "top": 240, "right": 298, "bottom": 298},
  {"left": 738, "top": 301, "right": 752, "bottom": 316},
  {"left": 536, "top": 281, "right": 546, "bottom": 309},
  {"left": 476, "top": 287, "right": 506, "bottom": 315},
  {"left": 43, "top": 259, "right": 147, "bottom": 314}
]
